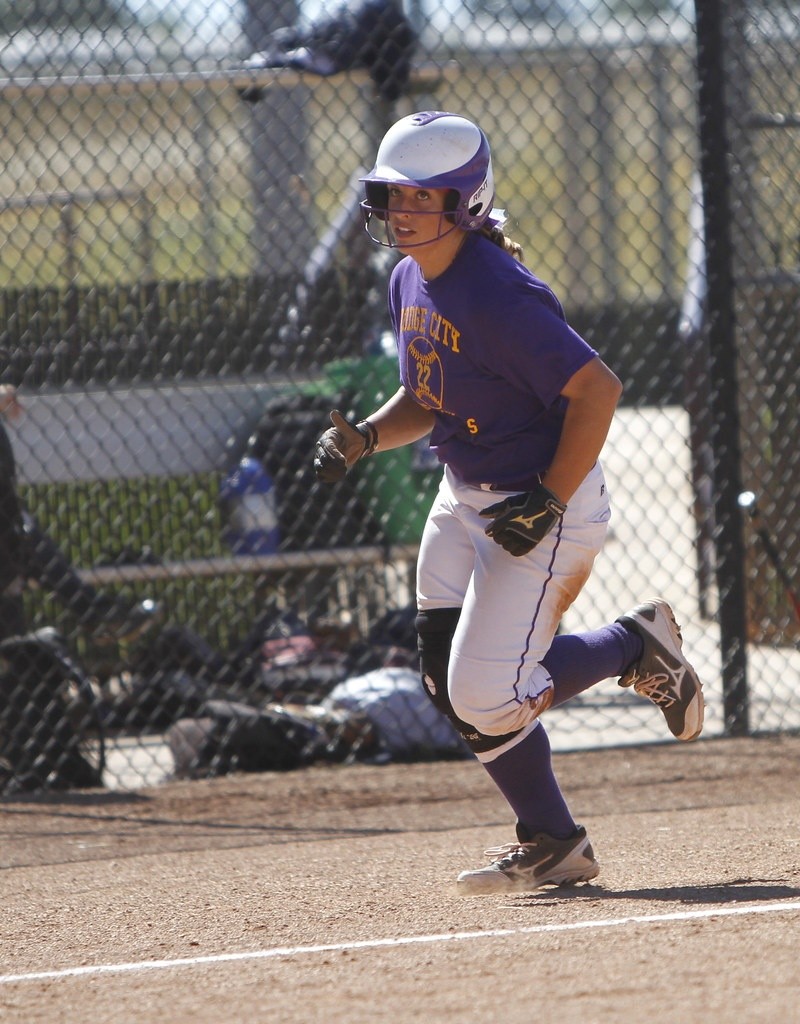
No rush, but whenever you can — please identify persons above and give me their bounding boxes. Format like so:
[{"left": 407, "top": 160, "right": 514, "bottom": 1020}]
[
  {"left": 314, "top": 111, "right": 704, "bottom": 898},
  {"left": 0, "top": 383, "right": 154, "bottom": 791},
  {"left": 226, "top": 385, "right": 393, "bottom": 657}
]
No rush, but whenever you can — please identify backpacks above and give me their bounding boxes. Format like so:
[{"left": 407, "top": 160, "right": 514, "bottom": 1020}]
[{"left": 0, "top": 625, "right": 107, "bottom": 793}]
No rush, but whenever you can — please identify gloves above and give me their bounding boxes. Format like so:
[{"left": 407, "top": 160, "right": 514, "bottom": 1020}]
[
  {"left": 314, "top": 408, "right": 380, "bottom": 485},
  {"left": 479, "top": 484, "right": 568, "bottom": 557}
]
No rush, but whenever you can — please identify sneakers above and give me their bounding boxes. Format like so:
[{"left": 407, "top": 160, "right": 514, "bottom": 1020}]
[
  {"left": 615, "top": 599, "right": 704, "bottom": 742},
  {"left": 458, "top": 815, "right": 600, "bottom": 896}
]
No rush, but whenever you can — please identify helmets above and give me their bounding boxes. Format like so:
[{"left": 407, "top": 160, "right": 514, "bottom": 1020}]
[{"left": 358, "top": 108, "right": 495, "bottom": 230}]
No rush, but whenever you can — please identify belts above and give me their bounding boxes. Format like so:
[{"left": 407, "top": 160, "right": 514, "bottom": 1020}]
[{"left": 474, "top": 470, "right": 549, "bottom": 492}]
